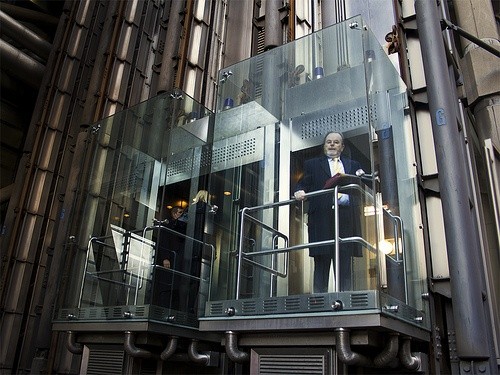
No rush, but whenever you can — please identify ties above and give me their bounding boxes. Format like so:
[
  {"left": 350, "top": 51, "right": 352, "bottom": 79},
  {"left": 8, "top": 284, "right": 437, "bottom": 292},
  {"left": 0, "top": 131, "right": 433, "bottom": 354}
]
[{"left": 334, "top": 158, "right": 343, "bottom": 200}]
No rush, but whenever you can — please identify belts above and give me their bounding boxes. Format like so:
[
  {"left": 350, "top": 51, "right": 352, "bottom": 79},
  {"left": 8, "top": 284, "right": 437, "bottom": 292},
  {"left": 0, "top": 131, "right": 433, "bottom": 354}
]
[{"left": 332, "top": 204, "right": 350, "bottom": 209}]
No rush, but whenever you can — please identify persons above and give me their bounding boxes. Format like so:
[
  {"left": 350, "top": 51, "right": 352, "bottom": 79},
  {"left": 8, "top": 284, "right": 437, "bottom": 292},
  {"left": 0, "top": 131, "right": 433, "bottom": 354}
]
[
  {"left": 152, "top": 207, "right": 184, "bottom": 307},
  {"left": 295, "top": 132, "right": 365, "bottom": 293},
  {"left": 174, "top": 189, "right": 213, "bottom": 313}
]
